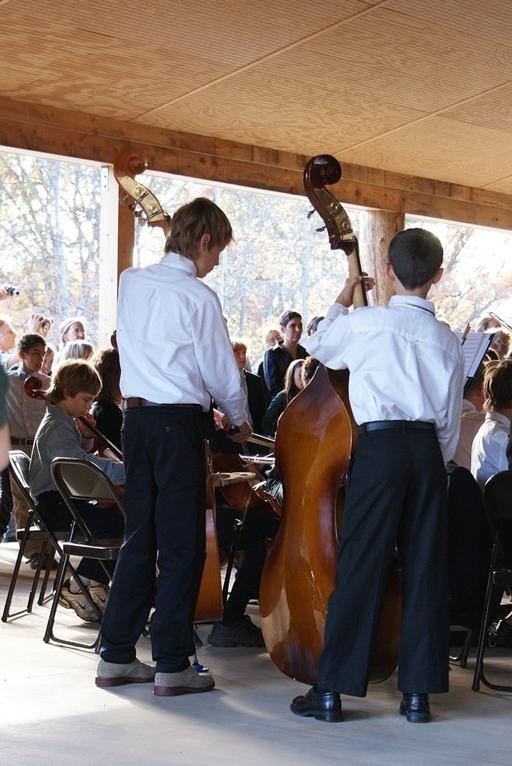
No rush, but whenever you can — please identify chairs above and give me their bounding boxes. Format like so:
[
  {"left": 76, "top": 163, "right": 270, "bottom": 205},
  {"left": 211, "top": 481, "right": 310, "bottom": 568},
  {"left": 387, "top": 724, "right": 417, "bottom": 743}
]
[
  {"left": 0, "top": 448, "right": 104, "bottom": 629},
  {"left": 472, "top": 470, "right": 512, "bottom": 694},
  {"left": 443, "top": 461, "right": 494, "bottom": 668},
  {"left": 42, "top": 456, "right": 204, "bottom": 653}
]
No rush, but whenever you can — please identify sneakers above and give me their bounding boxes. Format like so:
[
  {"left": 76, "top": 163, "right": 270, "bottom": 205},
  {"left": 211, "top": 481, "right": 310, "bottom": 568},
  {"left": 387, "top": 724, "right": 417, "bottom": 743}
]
[
  {"left": 154, "top": 665, "right": 215, "bottom": 696},
  {"left": 25, "top": 554, "right": 44, "bottom": 570},
  {"left": 90, "top": 584, "right": 111, "bottom": 612},
  {"left": 58, "top": 578, "right": 103, "bottom": 623},
  {"left": 95, "top": 658, "right": 156, "bottom": 687},
  {"left": 42, "top": 555, "right": 59, "bottom": 570},
  {"left": 207, "top": 614, "right": 265, "bottom": 648}
]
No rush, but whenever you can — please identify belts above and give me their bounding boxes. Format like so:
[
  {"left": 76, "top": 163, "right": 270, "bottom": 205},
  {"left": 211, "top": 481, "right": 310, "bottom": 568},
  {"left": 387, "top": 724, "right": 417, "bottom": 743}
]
[
  {"left": 11, "top": 438, "right": 33, "bottom": 446},
  {"left": 122, "top": 397, "right": 202, "bottom": 411},
  {"left": 357, "top": 419, "right": 437, "bottom": 435}
]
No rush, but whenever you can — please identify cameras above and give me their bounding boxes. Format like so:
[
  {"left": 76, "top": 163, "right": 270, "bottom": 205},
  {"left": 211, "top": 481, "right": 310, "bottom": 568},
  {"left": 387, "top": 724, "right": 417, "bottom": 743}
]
[
  {"left": 5, "top": 285, "right": 22, "bottom": 300},
  {"left": 40, "top": 316, "right": 51, "bottom": 328}
]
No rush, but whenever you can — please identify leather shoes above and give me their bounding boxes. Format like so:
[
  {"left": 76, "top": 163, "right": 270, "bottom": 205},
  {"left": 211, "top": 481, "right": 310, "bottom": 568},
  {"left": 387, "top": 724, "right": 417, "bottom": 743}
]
[
  {"left": 290, "top": 683, "right": 343, "bottom": 722},
  {"left": 4, "top": 537, "right": 17, "bottom": 542},
  {"left": 400, "top": 693, "right": 430, "bottom": 723}
]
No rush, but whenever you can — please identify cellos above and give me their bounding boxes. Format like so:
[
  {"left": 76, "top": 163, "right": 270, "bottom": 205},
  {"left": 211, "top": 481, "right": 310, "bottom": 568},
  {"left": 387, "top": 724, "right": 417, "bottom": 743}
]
[
  {"left": 261, "top": 154, "right": 403, "bottom": 689},
  {"left": 113, "top": 146, "right": 226, "bottom": 627},
  {"left": 22, "top": 376, "right": 124, "bottom": 511}
]
